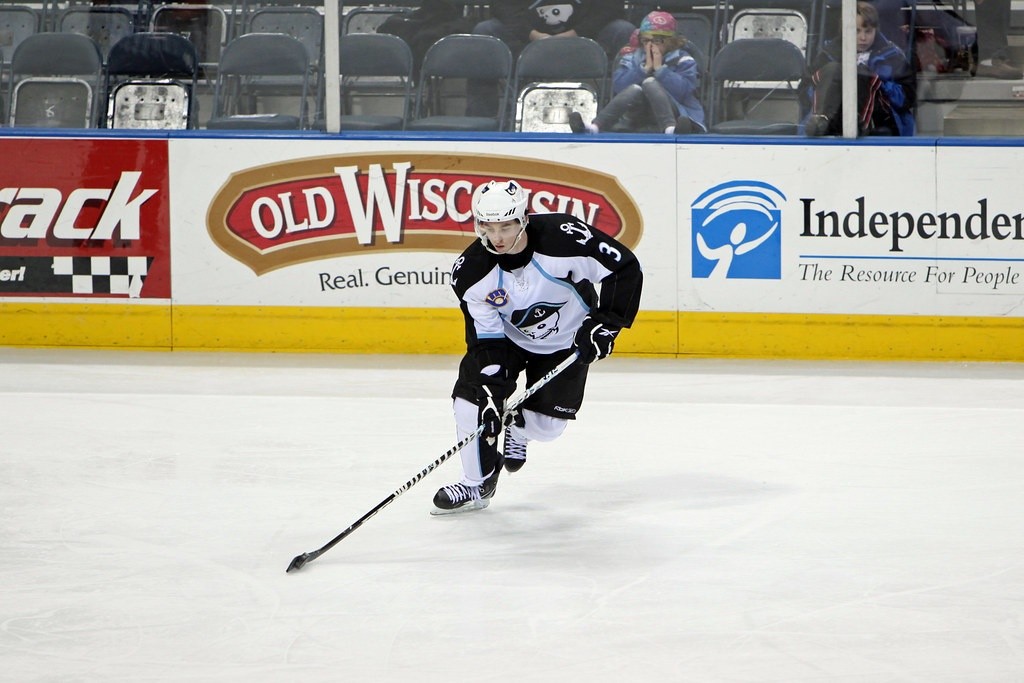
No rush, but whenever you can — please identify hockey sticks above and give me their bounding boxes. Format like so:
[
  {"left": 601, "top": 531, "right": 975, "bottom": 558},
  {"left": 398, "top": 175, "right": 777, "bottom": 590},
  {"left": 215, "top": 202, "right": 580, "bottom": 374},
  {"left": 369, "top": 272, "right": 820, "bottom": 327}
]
[{"left": 286, "top": 349, "right": 578, "bottom": 570}]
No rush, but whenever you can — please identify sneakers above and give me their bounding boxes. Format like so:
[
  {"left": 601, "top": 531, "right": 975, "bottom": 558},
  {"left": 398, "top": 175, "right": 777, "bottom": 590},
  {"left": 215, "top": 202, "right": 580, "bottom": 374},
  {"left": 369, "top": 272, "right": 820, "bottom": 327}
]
[
  {"left": 503, "top": 405, "right": 529, "bottom": 475},
  {"left": 430, "top": 451, "right": 504, "bottom": 517}
]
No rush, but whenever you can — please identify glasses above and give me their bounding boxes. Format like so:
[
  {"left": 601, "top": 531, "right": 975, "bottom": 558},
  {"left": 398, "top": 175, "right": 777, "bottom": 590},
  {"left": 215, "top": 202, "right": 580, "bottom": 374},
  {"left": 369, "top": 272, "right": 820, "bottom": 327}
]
[{"left": 640, "top": 36, "right": 671, "bottom": 45}]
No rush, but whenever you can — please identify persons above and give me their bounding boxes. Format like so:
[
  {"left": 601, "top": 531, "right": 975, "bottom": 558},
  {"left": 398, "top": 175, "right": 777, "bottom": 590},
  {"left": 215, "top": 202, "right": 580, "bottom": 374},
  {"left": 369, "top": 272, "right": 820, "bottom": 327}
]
[
  {"left": 466, "top": 0, "right": 637, "bottom": 117},
  {"left": 569, "top": 12, "right": 708, "bottom": 133},
  {"left": 429, "top": 180, "right": 643, "bottom": 515},
  {"left": 878, "top": 0, "right": 1023, "bottom": 78},
  {"left": 799, "top": 3, "right": 916, "bottom": 138}
]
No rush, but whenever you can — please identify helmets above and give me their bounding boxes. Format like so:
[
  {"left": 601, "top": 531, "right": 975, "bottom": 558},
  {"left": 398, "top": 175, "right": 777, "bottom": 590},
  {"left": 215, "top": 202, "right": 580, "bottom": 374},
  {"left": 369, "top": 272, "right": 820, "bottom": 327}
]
[{"left": 471, "top": 180, "right": 527, "bottom": 254}]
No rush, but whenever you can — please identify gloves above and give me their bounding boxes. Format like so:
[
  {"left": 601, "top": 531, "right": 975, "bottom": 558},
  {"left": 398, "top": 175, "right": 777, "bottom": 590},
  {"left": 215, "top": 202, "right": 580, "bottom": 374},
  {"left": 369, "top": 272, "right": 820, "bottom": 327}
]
[
  {"left": 570, "top": 312, "right": 623, "bottom": 364},
  {"left": 475, "top": 383, "right": 519, "bottom": 447}
]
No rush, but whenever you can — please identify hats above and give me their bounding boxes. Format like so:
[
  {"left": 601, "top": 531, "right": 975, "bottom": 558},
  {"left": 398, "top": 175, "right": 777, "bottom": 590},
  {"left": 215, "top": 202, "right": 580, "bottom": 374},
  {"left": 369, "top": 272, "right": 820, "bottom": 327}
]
[{"left": 641, "top": 11, "right": 677, "bottom": 35}]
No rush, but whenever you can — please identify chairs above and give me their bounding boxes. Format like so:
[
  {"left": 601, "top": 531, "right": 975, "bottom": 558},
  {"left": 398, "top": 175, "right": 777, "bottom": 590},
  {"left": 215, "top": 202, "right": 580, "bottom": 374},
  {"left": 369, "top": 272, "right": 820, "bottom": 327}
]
[{"left": 0, "top": 0, "right": 1024, "bottom": 137}]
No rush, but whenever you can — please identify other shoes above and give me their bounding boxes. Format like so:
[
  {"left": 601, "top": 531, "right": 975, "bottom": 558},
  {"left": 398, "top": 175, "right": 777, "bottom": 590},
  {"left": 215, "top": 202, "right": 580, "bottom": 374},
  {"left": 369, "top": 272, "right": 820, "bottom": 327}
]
[
  {"left": 674, "top": 116, "right": 692, "bottom": 135},
  {"left": 571, "top": 111, "right": 595, "bottom": 134},
  {"left": 805, "top": 113, "right": 828, "bottom": 138}
]
